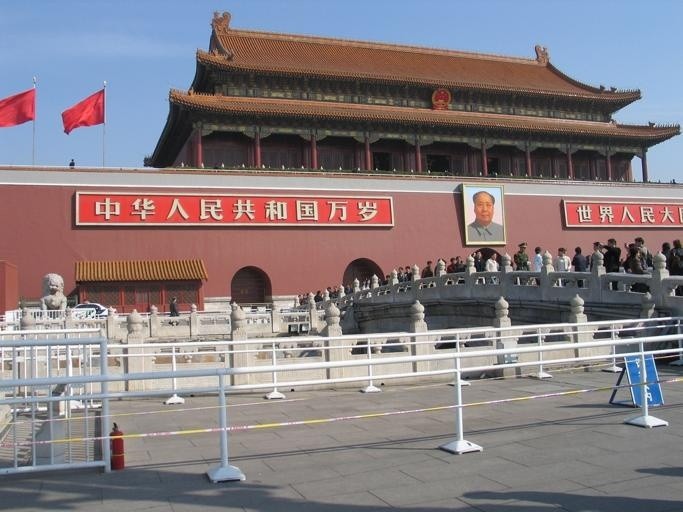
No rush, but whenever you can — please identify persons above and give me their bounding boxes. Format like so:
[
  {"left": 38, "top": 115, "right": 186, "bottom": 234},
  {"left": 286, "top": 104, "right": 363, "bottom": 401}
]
[
  {"left": 69, "top": 159, "right": 74, "bottom": 166},
  {"left": 468, "top": 191, "right": 502, "bottom": 242},
  {"left": 298, "top": 237, "right": 682, "bottom": 308},
  {"left": 168, "top": 297, "right": 180, "bottom": 325}
]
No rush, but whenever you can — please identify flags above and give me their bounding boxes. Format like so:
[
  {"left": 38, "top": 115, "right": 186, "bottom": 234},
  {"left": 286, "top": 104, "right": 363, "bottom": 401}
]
[
  {"left": 61, "top": 89, "right": 104, "bottom": 134},
  {"left": 0, "top": 87, "right": 35, "bottom": 128}
]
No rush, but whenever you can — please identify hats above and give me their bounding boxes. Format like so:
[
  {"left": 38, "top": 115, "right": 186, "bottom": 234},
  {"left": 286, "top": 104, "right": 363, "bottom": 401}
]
[{"left": 519, "top": 242, "right": 527, "bottom": 247}]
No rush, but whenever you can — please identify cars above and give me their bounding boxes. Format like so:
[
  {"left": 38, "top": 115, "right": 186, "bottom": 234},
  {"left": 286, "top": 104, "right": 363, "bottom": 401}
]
[{"left": 71, "top": 299, "right": 117, "bottom": 318}]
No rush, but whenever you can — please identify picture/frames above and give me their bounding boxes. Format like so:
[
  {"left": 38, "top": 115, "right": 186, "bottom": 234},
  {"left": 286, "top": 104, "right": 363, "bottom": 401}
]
[
  {"left": 563, "top": 200, "right": 683, "bottom": 228},
  {"left": 458, "top": 183, "right": 507, "bottom": 246},
  {"left": 76, "top": 190, "right": 395, "bottom": 226}
]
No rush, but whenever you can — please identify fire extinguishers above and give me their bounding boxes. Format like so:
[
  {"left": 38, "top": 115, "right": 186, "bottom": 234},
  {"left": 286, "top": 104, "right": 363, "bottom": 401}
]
[{"left": 110, "top": 423, "right": 124, "bottom": 470}]
